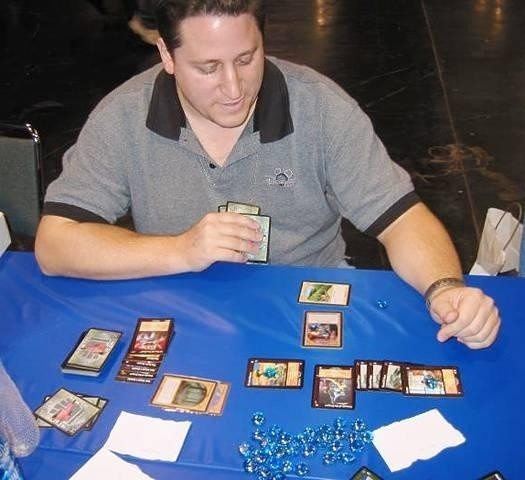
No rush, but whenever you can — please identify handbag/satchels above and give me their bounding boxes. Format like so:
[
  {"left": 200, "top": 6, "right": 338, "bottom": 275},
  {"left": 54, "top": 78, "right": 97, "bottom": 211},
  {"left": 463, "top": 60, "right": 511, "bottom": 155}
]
[{"left": 466, "top": 201, "right": 524, "bottom": 277}]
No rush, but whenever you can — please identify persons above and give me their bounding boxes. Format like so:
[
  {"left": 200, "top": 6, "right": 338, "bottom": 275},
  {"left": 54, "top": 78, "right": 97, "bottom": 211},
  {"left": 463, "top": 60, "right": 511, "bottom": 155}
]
[{"left": 33, "top": 1, "right": 502, "bottom": 351}]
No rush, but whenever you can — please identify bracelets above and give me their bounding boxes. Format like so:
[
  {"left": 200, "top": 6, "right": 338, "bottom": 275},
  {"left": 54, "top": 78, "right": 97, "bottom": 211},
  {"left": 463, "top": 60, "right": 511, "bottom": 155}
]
[{"left": 423, "top": 277, "right": 470, "bottom": 312}]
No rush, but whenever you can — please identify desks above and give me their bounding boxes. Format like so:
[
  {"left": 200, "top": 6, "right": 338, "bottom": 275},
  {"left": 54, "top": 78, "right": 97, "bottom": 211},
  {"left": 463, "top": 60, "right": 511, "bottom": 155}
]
[{"left": 0, "top": 252, "right": 525, "bottom": 480}]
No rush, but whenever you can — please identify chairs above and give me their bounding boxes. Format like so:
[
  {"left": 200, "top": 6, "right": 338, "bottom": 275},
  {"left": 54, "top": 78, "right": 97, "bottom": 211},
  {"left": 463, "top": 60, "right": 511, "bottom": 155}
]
[{"left": 0, "top": 122, "right": 45, "bottom": 252}]
